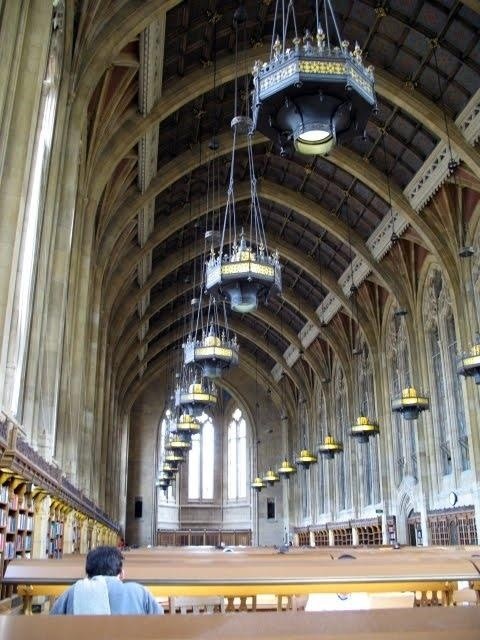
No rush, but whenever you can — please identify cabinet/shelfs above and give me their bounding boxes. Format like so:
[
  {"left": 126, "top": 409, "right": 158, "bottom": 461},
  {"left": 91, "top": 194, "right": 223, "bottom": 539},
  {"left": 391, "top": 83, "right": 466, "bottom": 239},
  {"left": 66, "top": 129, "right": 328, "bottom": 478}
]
[
  {"left": 0, "top": 441, "right": 118, "bottom": 559},
  {"left": 294, "top": 504, "right": 477, "bottom": 546}
]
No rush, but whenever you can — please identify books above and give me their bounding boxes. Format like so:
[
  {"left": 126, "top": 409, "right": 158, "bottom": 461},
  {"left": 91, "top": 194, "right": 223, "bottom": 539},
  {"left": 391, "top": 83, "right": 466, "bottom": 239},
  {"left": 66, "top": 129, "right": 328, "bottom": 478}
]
[{"left": 0, "top": 484, "right": 87, "bottom": 602}]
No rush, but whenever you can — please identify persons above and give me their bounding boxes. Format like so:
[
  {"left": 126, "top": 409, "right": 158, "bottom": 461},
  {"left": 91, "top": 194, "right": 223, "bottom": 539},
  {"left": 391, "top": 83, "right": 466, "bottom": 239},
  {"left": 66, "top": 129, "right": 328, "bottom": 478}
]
[{"left": 48, "top": 545, "right": 165, "bottom": 616}]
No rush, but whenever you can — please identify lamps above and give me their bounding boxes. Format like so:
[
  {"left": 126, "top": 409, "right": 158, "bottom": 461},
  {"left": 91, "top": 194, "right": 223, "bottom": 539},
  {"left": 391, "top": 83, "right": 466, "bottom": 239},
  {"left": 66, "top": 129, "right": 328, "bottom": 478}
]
[
  {"left": 343, "top": 189, "right": 381, "bottom": 445},
  {"left": 426, "top": 36, "right": 480, "bottom": 386},
  {"left": 202, "top": 1, "right": 285, "bottom": 314},
  {"left": 294, "top": 273, "right": 317, "bottom": 470},
  {"left": 153, "top": 316, "right": 278, "bottom": 495},
  {"left": 249, "top": 1, "right": 380, "bottom": 161},
  {"left": 376, "top": 120, "right": 433, "bottom": 421},
  {"left": 278, "top": 296, "right": 296, "bottom": 484},
  {"left": 316, "top": 234, "right": 346, "bottom": 462}
]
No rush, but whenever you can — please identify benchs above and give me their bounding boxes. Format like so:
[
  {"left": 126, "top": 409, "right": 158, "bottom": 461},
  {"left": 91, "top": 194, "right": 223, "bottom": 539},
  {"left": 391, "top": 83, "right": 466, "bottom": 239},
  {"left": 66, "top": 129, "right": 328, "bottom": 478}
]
[{"left": 1, "top": 544, "right": 480, "bottom": 640}]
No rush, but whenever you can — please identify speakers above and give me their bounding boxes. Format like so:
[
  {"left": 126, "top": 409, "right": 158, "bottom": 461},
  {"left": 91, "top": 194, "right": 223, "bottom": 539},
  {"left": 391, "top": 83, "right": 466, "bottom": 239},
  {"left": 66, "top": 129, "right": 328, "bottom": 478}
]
[
  {"left": 135, "top": 500, "right": 141, "bottom": 518},
  {"left": 268, "top": 502, "right": 274, "bottom": 518}
]
